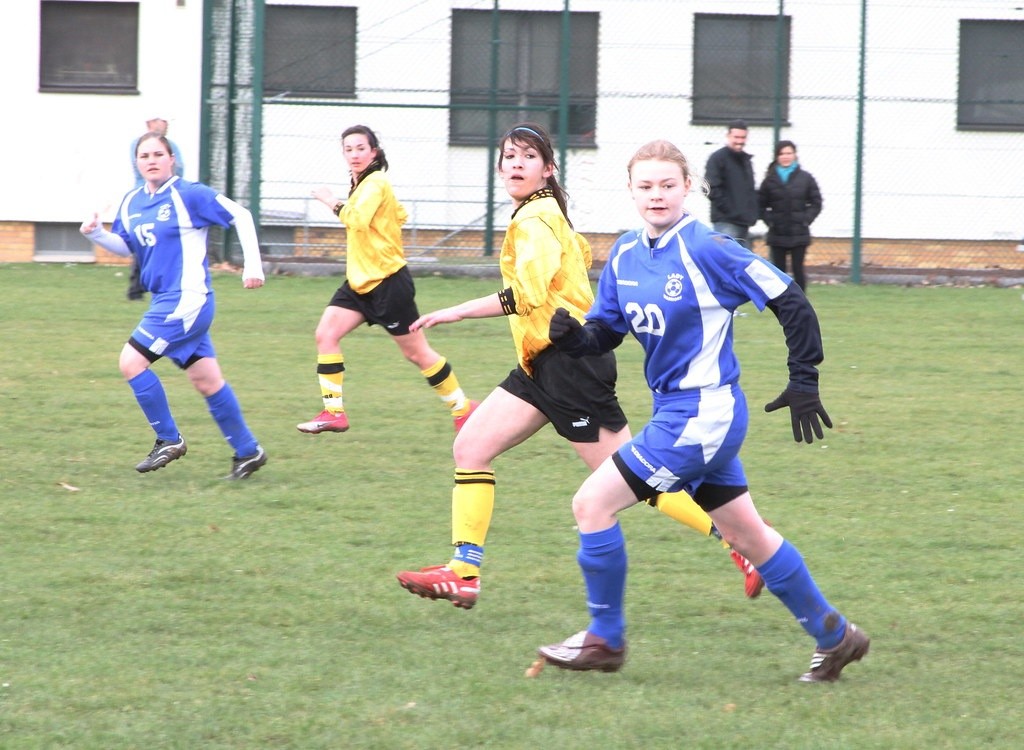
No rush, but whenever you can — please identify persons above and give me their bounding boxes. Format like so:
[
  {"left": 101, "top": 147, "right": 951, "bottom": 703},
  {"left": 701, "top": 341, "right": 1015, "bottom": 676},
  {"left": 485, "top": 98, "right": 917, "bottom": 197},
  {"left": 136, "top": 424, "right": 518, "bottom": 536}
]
[
  {"left": 78, "top": 131, "right": 268, "bottom": 479},
  {"left": 757, "top": 140, "right": 823, "bottom": 293},
  {"left": 127, "top": 117, "right": 184, "bottom": 300},
  {"left": 539, "top": 140, "right": 872, "bottom": 683},
  {"left": 296, "top": 125, "right": 479, "bottom": 435},
  {"left": 396, "top": 125, "right": 764, "bottom": 610},
  {"left": 702, "top": 120, "right": 757, "bottom": 252}
]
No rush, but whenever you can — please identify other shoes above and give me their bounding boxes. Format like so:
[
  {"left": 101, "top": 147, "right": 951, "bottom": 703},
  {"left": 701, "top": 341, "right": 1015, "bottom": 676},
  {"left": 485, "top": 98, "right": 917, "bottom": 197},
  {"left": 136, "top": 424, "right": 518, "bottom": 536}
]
[{"left": 127, "top": 286, "right": 145, "bottom": 300}]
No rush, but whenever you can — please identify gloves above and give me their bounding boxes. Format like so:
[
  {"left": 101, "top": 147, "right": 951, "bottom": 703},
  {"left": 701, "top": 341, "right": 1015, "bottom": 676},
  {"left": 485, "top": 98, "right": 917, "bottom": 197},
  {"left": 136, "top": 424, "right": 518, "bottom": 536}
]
[
  {"left": 548, "top": 308, "right": 588, "bottom": 358},
  {"left": 764, "top": 388, "right": 832, "bottom": 444}
]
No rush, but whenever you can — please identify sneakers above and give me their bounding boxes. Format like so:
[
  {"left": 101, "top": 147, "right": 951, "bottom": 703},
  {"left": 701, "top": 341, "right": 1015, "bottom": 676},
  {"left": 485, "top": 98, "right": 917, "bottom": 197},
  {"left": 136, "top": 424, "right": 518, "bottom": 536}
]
[
  {"left": 798, "top": 621, "right": 871, "bottom": 682},
  {"left": 396, "top": 565, "right": 482, "bottom": 609},
  {"left": 538, "top": 631, "right": 626, "bottom": 674},
  {"left": 296, "top": 409, "right": 350, "bottom": 434},
  {"left": 223, "top": 444, "right": 268, "bottom": 481},
  {"left": 729, "top": 517, "right": 773, "bottom": 599},
  {"left": 454, "top": 401, "right": 479, "bottom": 434},
  {"left": 136, "top": 433, "right": 186, "bottom": 473}
]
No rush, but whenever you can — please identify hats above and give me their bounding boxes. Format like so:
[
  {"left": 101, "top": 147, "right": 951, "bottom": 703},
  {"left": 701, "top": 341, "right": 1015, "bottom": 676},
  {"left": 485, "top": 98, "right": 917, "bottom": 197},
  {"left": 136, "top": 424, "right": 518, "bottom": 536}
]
[{"left": 144, "top": 113, "right": 171, "bottom": 122}]
